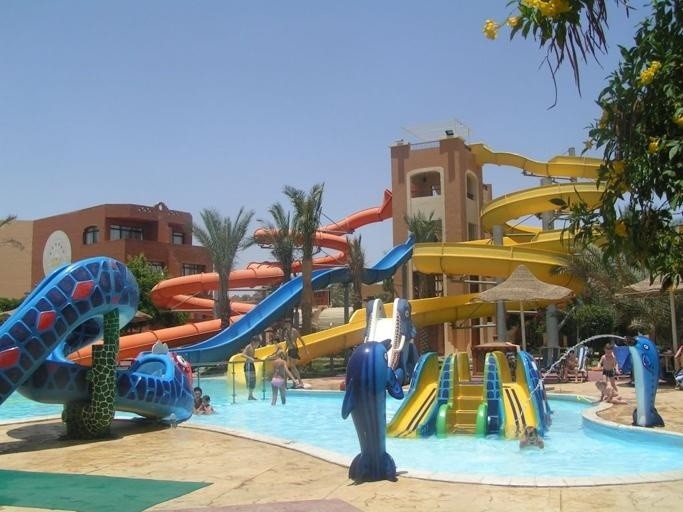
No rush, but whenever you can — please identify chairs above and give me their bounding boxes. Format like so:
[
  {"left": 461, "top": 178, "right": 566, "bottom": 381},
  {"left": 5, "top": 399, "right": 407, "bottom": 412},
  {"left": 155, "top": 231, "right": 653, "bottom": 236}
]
[{"left": 537, "top": 346, "right": 589, "bottom": 384}]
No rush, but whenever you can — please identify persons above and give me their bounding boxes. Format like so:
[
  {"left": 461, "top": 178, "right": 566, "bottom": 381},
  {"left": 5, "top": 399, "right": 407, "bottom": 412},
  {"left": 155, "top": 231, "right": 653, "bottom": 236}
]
[
  {"left": 270, "top": 350, "right": 299, "bottom": 405},
  {"left": 283, "top": 318, "right": 309, "bottom": 388},
  {"left": 564, "top": 351, "right": 580, "bottom": 384},
  {"left": 194, "top": 387, "right": 203, "bottom": 415},
  {"left": 596, "top": 344, "right": 621, "bottom": 401},
  {"left": 197, "top": 396, "right": 214, "bottom": 417},
  {"left": 240, "top": 336, "right": 262, "bottom": 400}
]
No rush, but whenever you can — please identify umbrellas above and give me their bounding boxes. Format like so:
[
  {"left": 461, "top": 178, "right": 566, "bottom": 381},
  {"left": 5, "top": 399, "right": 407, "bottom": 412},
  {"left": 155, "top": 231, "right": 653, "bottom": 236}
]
[
  {"left": 468, "top": 263, "right": 573, "bottom": 350},
  {"left": 612, "top": 272, "right": 682, "bottom": 349}
]
